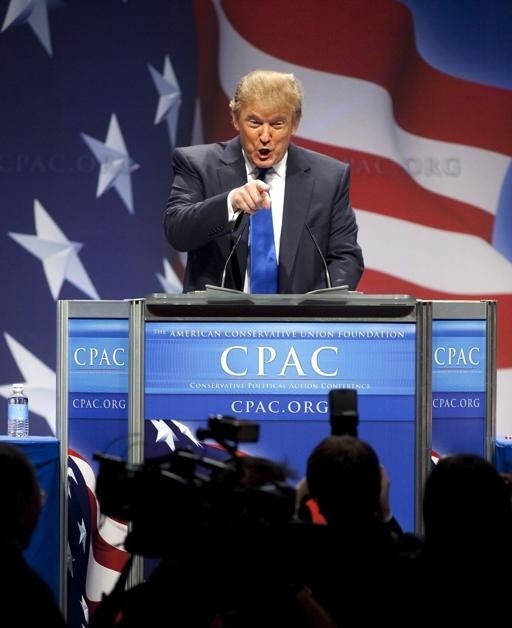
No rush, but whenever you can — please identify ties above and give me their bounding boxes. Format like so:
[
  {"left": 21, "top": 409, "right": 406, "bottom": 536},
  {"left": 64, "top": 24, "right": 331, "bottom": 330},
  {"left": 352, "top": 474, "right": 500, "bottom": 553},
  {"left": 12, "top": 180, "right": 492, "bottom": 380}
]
[{"left": 249, "top": 168, "right": 279, "bottom": 294}]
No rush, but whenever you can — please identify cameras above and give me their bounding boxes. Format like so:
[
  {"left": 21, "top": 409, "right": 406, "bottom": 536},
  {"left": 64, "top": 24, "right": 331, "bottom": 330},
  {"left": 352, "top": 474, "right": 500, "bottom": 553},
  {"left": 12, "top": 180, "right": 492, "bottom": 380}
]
[{"left": 329, "top": 389, "right": 358, "bottom": 438}]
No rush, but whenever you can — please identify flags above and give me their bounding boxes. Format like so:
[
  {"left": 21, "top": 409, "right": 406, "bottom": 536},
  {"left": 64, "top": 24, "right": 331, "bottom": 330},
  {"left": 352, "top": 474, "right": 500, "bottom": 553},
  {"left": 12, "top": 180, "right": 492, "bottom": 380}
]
[{"left": 0, "top": 0, "right": 512, "bottom": 439}]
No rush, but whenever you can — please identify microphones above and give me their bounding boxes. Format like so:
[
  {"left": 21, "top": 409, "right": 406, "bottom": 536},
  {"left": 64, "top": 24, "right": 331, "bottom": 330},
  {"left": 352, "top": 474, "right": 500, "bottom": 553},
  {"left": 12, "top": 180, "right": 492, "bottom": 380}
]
[
  {"left": 205, "top": 214, "right": 250, "bottom": 295},
  {"left": 303, "top": 217, "right": 349, "bottom": 295}
]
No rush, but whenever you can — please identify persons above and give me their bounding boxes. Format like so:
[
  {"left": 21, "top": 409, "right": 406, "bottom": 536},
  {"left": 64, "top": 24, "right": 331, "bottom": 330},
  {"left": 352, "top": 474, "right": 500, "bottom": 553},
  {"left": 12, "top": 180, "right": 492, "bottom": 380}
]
[
  {"left": 163, "top": 67, "right": 364, "bottom": 293},
  {"left": 134, "top": 433, "right": 511, "bottom": 628},
  {"left": 0, "top": 442, "right": 67, "bottom": 628}
]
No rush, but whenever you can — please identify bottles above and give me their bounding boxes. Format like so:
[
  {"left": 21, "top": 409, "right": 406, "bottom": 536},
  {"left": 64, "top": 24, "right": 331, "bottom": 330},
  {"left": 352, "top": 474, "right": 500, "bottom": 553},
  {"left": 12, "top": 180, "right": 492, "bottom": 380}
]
[{"left": 7, "top": 384, "right": 29, "bottom": 439}]
